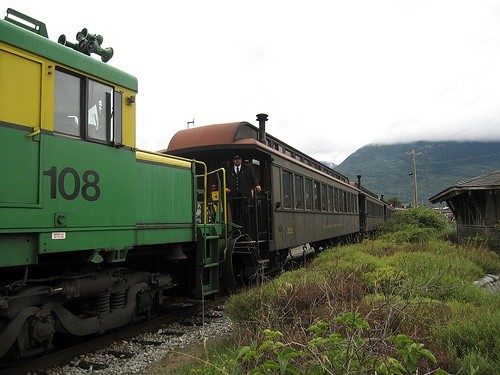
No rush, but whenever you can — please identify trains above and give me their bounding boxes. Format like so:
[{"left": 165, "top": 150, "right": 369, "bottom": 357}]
[{"left": 0, "top": 8, "right": 396, "bottom": 366}]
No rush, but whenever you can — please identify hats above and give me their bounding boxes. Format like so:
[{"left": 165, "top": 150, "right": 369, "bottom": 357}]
[{"left": 231, "top": 152, "right": 242, "bottom": 159}]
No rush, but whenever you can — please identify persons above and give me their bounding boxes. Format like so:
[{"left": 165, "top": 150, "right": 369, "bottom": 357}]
[{"left": 226, "top": 154, "right": 261, "bottom": 233}]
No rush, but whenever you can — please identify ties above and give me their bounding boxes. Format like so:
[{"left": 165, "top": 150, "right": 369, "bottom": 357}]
[{"left": 237, "top": 167, "right": 239, "bottom": 173}]
